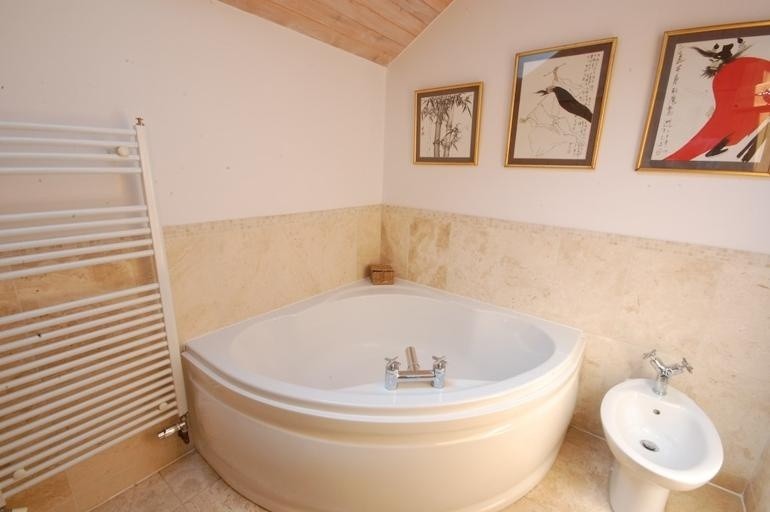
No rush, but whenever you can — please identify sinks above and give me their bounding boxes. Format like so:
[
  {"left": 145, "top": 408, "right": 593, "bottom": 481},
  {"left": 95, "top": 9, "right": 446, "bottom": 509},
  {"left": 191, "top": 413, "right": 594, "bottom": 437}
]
[{"left": 600, "top": 378, "right": 724, "bottom": 512}]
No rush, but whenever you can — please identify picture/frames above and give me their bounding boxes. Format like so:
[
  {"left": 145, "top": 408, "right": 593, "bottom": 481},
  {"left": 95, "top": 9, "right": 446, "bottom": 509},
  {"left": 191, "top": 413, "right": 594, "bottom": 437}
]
[
  {"left": 411, "top": 84, "right": 482, "bottom": 165},
  {"left": 635, "top": 19, "right": 769, "bottom": 174},
  {"left": 505, "top": 35, "right": 618, "bottom": 168}
]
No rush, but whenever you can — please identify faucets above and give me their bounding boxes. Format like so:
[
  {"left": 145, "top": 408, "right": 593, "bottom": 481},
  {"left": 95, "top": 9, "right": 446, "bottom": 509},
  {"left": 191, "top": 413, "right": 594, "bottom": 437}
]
[
  {"left": 405, "top": 347, "right": 419, "bottom": 371},
  {"left": 654, "top": 361, "right": 672, "bottom": 396}
]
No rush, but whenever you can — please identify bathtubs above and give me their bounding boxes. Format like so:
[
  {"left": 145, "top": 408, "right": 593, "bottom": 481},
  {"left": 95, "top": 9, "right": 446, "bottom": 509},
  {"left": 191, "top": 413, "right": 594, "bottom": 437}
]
[{"left": 179, "top": 277, "right": 588, "bottom": 512}]
